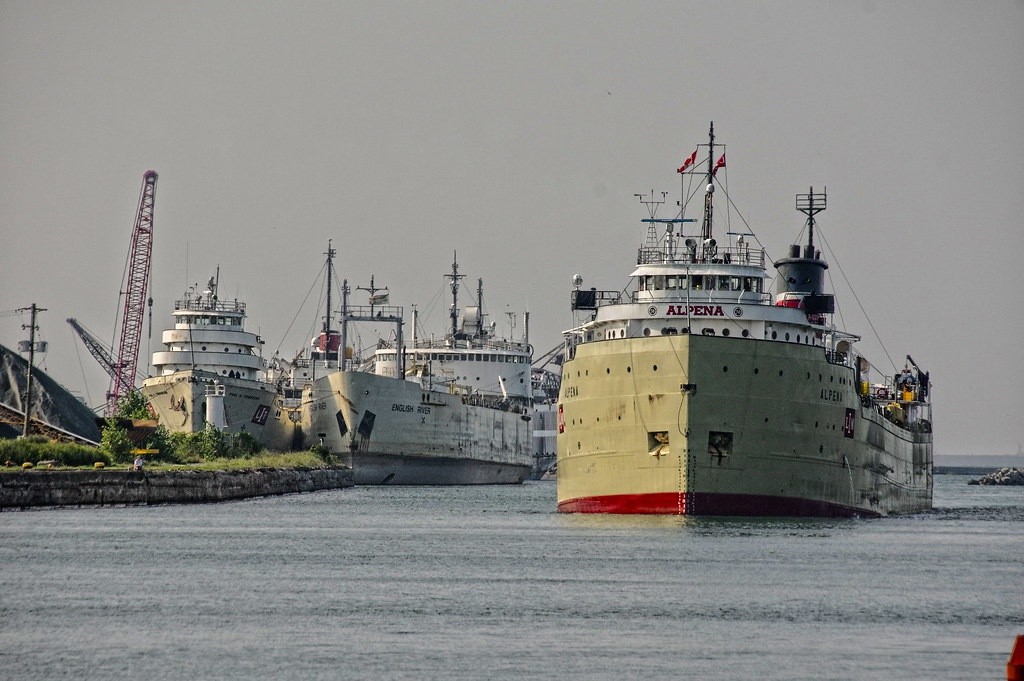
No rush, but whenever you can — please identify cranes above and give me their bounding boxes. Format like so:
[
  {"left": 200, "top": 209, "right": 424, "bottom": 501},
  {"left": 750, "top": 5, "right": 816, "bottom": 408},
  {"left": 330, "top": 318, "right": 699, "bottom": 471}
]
[{"left": 67, "top": 170, "right": 157, "bottom": 419}]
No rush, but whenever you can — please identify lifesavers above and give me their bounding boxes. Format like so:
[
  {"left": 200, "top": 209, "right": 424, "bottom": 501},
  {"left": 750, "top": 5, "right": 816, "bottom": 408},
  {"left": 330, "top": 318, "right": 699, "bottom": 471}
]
[{"left": 877, "top": 387, "right": 888, "bottom": 398}]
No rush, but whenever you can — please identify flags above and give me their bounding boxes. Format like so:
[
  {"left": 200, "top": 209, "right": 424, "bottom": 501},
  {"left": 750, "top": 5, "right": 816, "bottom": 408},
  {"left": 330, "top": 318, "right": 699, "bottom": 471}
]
[
  {"left": 712, "top": 153, "right": 727, "bottom": 177},
  {"left": 676, "top": 149, "right": 698, "bottom": 173}
]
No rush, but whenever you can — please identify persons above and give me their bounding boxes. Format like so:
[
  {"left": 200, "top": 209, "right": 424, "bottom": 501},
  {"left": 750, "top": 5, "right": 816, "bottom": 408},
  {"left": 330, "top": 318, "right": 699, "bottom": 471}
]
[{"left": 134, "top": 456, "right": 143, "bottom": 470}]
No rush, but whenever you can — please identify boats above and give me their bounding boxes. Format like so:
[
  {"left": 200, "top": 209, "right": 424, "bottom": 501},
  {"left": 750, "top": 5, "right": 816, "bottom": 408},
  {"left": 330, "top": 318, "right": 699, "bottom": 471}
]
[
  {"left": 142, "top": 238, "right": 560, "bottom": 485},
  {"left": 556, "top": 121, "right": 934, "bottom": 515}
]
